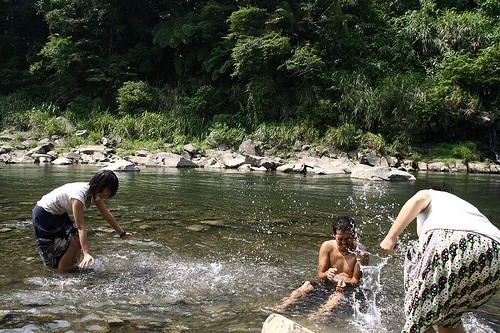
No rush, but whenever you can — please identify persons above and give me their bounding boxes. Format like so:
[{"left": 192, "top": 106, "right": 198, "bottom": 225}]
[
  {"left": 379, "top": 184, "right": 500, "bottom": 333},
  {"left": 261, "top": 217, "right": 368, "bottom": 324},
  {"left": 32, "top": 169, "right": 133, "bottom": 272}
]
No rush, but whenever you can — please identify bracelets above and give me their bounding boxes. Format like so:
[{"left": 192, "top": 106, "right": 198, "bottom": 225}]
[{"left": 119, "top": 230, "right": 126, "bottom": 238}]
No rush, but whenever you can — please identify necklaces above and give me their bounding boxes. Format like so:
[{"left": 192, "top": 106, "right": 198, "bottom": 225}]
[{"left": 337, "top": 243, "right": 350, "bottom": 256}]
